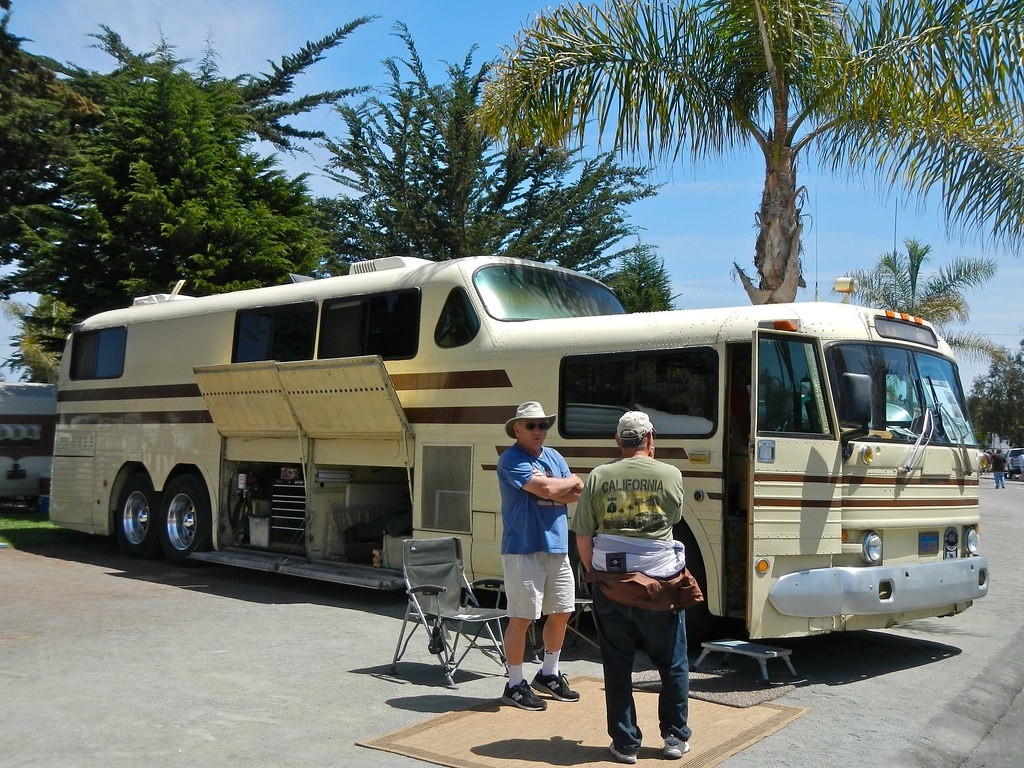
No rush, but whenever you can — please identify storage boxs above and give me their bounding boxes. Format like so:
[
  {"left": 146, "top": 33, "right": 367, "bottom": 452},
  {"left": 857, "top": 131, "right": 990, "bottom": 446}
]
[
  {"left": 38, "top": 494, "right": 50, "bottom": 514},
  {"left": 39, "top": 473, "right": 50, "bottom": 495},
  {"left": 249, "top": 517, "right": 269, "bottom": 548}
]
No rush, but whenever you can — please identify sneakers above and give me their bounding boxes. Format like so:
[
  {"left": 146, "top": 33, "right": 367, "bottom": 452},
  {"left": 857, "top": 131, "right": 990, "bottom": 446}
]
[
  {"left": 664, "top": 737, "right": 691, "bottom": 758},
  {"left": 609, "top": 742, "right": 637, "bottom": 763},
  {"left": 502, "top": 679, "right": 547, "bottom": 711},
  {"left": 530, "top": 669, "right": 580, "bottom": 701}
]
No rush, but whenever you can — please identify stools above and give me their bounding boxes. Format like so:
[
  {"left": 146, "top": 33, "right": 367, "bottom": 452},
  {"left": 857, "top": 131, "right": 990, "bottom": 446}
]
[{"left": 688, "top": 638, "right": 799, "bottom": 685}]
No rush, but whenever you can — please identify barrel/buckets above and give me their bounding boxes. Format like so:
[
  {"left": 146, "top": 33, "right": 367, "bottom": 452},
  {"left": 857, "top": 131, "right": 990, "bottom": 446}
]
[
  {"left": 238, "top": 473, "right": 247, "bottom": 489},
  {"left": 251, "top": 500, "right": 271, "bottom": 517},
  {"left": 247, "top": 515, "right": 270, "bottom": 547}
]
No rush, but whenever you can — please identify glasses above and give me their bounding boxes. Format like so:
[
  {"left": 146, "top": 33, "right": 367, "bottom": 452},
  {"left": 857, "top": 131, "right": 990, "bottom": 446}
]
[{"left": 517, "top": 422, "right": 549, "bottom": 431}]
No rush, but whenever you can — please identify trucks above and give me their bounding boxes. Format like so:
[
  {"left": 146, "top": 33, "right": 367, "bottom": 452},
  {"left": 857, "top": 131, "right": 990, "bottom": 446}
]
[{"left": 48, "top": 257, "right": 988, "bottom": 638}]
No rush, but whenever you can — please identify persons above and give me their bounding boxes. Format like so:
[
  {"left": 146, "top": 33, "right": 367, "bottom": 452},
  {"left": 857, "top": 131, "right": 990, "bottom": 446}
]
[
  {"left": 979, "top": 449, "right": 1024, "bottom": 489},
  {"left": 571, "top": 411, "right": 692, "bottom": 763},
  {"left": 497, "top": 400, "right": 584, "bottom": 711}
]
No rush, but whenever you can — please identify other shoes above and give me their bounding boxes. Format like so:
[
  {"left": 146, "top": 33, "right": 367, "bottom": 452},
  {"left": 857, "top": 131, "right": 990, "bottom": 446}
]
[
  {"left": 995, "top": 487, "right": 999, "bottom": 489},
  {"left": 1003, "top": 487, "right": 1005, "bottom": 489}
]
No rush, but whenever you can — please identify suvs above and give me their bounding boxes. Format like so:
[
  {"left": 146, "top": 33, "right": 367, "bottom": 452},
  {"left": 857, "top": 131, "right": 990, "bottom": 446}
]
[{"left": 1003, "top": 448, "right": 1024, "bottom": 479}]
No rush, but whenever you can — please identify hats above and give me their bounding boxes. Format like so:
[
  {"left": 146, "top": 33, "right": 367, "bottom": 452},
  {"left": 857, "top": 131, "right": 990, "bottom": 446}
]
[
  {"left": 616, "top": 411, "right": 658, "bottom": 440},
  {"left": 505, "top": 402, "right": 557, "bottom": 439}
]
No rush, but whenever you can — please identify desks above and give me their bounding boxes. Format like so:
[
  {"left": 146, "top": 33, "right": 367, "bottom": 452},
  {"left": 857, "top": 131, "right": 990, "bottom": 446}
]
[{"left": 537, "top": 598, "right": 601, "bottom": 652}]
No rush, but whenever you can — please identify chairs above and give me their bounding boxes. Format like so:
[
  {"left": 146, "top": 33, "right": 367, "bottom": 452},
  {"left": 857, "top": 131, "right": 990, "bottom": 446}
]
[{"left": 386, "top": 537, "right": 509, "bottom": 691}]
